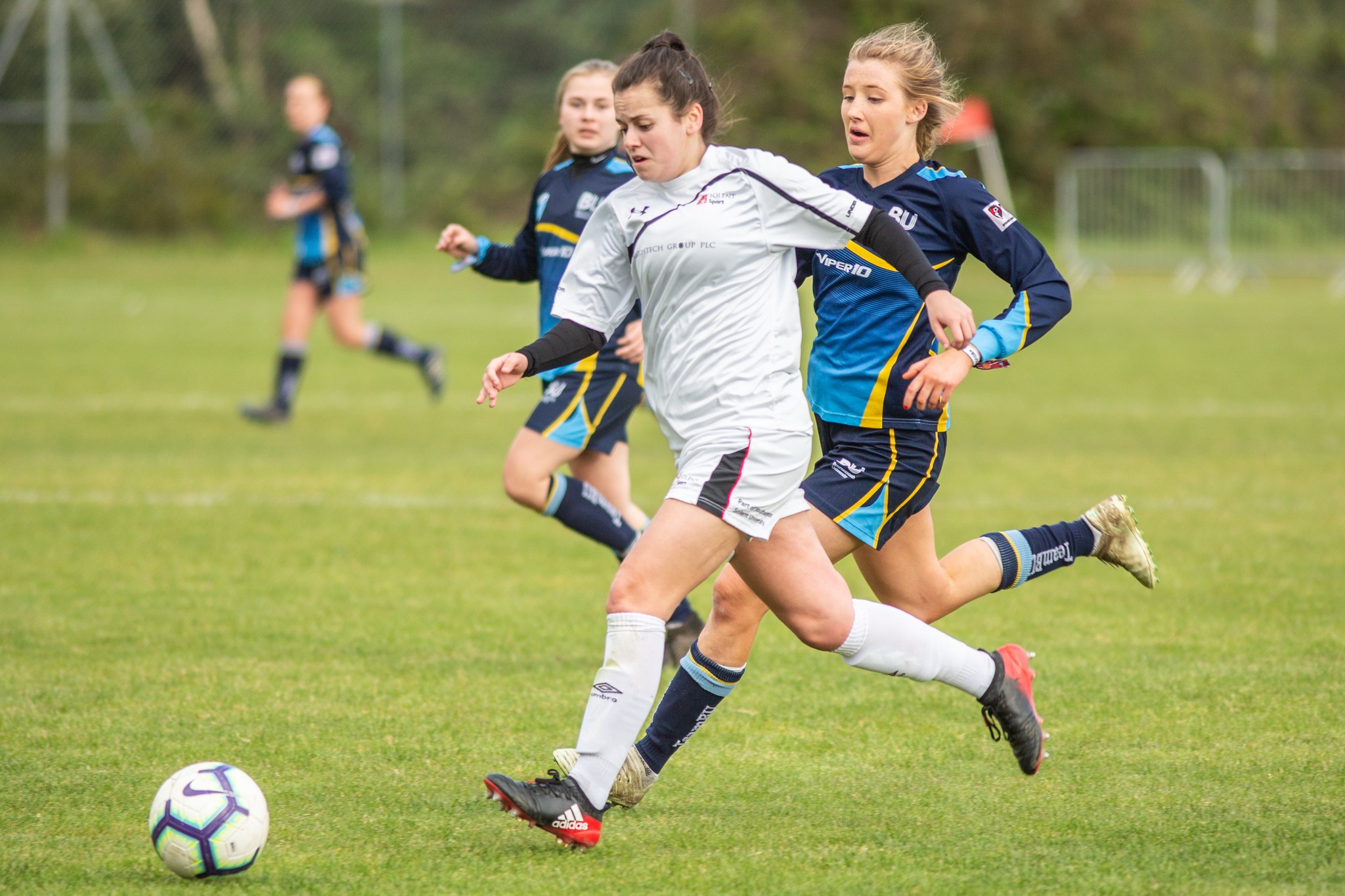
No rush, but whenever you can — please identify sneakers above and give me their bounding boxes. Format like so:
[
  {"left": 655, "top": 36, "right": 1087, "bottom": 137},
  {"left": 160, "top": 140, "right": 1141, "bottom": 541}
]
[
  {"left": 555, "top": 743, "right": 655, "bottom": 814},
  {"left": 482, "top": 770, "right": 613, "bottom": 852},
  {"left": 975, "top": 644, "right": 1051, "bottom": 776},
  {"left": 1085, "top": 494, "right": 1161, "bottom": 590}
]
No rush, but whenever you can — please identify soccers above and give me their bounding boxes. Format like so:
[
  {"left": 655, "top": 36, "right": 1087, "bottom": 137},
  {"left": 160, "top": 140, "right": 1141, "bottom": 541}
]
[{"left": 148, "top": 761, "right": 269, "bottom": 883}]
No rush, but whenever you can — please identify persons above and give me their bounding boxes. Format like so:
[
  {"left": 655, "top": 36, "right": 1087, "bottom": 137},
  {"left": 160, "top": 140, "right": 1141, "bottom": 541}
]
[
  {"left": 477, "top": 30, "right": 1049, "bottom": 847},
  {"left": 552, "top": 21, "right": 1161, "bottom": 813},
  {"left": 436, "top": 60, "right": 706, "bottom": 667},
  {"left": 237, "top": 72, "right": 446, "bottom": 425}
]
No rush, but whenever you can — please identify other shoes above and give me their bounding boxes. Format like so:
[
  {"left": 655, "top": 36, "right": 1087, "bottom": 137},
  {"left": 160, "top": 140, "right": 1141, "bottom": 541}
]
[
  {"left": 623, "top": 530, "right": 693, "bottom": 628},
  {"left": 420, "top": 349, "right": 445, "bottom": 391},
  {"left": 241, "top": 398, "right": 294, "bottom": 427}
]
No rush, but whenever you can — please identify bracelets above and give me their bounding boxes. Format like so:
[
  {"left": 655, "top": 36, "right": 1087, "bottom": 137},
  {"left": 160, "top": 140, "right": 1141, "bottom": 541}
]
[{"left": 963, "top": 346, "right": 981, "bottom": 367}]
